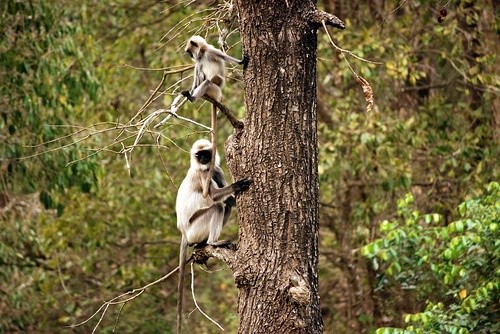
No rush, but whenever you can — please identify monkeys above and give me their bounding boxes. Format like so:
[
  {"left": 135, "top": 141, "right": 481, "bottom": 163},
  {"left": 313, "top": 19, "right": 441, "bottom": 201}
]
[
  {"left": 181, "top": 35, "right": 247, "bottom": 199},
  {"left": 176, "top": 138, "right": 253, "bottom": 334}
]
